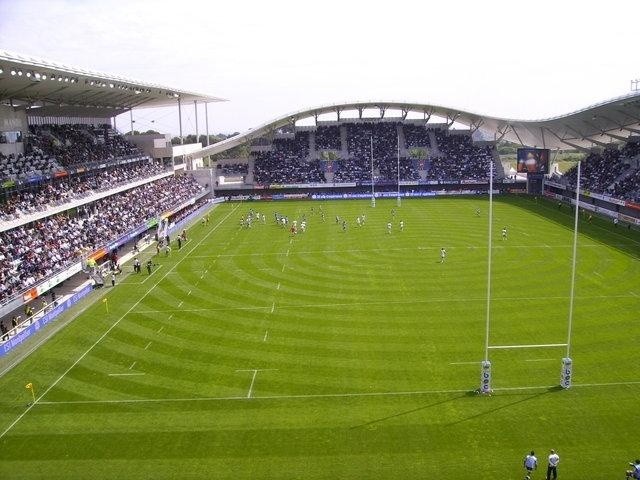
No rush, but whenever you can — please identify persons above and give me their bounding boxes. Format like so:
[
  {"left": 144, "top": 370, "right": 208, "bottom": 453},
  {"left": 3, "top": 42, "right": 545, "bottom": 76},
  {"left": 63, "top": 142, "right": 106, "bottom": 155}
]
[
  {"left": 440, "top": 248, "right": 446, "bottom": 264},
  {"left": 546, "top": 449, "right": 559, "bottom": 480},
  {"left": 532, "top": 134, "right": 640, "bottom": 232},
  {"left": 0, "top": 121, "right": 210, "bottom": 335},
  {"left": 629, "top": 458, "right": 640, "bottom": 480},
  {"left": 216, "top": 121, "right": 509, "bottom": 199},
  {"left": 240, "top": 205, "right": 404, "bottom": 237},
  {"left": 502, "top": 228, "right": 507, "bottom": 241},
  {"left": 524, "top": 451, "right": 537, "bottom": 480}
]
